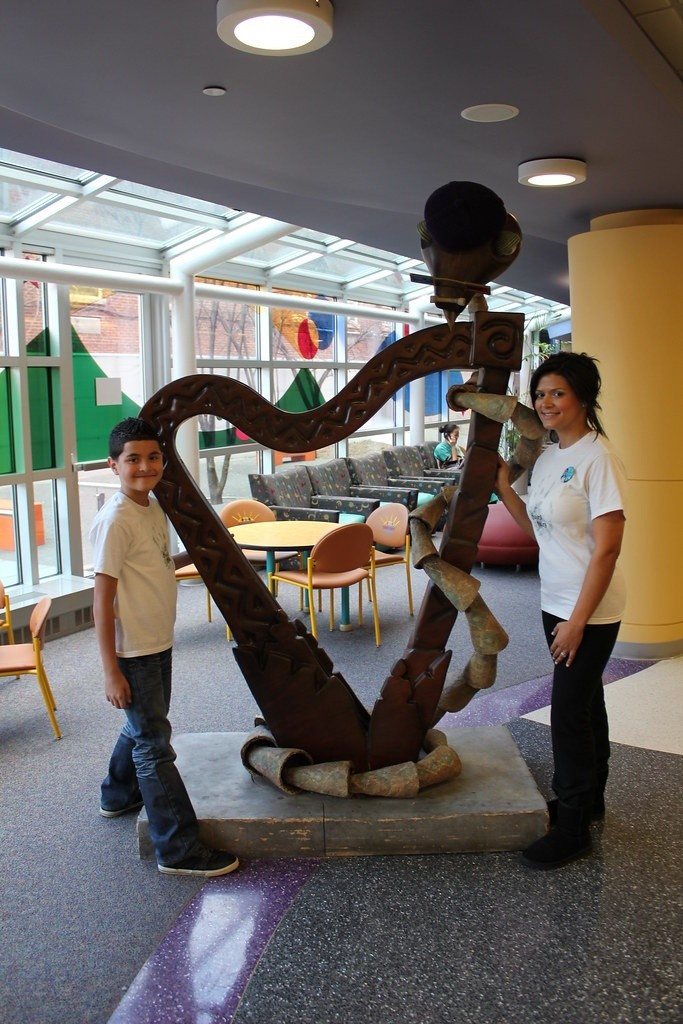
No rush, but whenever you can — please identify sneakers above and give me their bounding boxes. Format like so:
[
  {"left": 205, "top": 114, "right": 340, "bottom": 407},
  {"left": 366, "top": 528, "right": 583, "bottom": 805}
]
[{"left": 98, "top": 787, "right": 239, "bottom": 876}]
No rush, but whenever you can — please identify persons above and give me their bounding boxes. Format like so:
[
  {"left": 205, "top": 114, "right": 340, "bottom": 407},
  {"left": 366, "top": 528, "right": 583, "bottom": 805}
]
[
  {"left": 89, "top": 418, "right": 239, "bottom": 876},
  {"left": 434, "top": 424, "right": 499, "bottom": 505},
  {"left": 490, "top": 350, "right": 626, "bottom": 870}
]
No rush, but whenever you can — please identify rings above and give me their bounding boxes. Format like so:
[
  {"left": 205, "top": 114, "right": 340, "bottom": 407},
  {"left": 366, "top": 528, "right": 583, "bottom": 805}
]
[{"left": 560, "top": 651, "right": 566, "bottom": 658}]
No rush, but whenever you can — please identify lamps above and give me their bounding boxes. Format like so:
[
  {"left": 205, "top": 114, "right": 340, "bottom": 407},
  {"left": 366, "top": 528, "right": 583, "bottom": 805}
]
[
  {"left": 516, "top": 157, "right": 589, "bottom": 189},
  {"left": 215, "top": 0, "right": 336, "bottom": 57}
]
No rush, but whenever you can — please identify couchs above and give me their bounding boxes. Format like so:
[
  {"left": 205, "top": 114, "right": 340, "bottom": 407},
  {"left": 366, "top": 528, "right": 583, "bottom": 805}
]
[
  {"left": 340, "top": 450, "right": 446, "bottom": 536},
  {"left": 383, "top": 445, "right": 462, "bottom": 521},
  {"left": 298, "top": 458, "right": 411, "bottom": 553},
  {"left": 248, "top": 466, "right": 381, "bottom": 571},
  {"left": 415, "top": 441, "right": 442, "bottom": 471}
]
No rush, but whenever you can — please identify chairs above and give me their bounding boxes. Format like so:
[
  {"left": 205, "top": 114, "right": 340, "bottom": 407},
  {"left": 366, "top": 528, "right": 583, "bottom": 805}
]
[
  {"left": 218, "top": 499, "right": 304, "bottom": 597},
  {"left": 317, "top": 502, "right": 414, "bottom": 628},
  {"left": 174, "top": 561, "right": 213, "bottom": 623},
  {"left": 0, "top": 595, "right": 63, "bottom": 740},
  {"left": 1, "top": 582, "right": 21, "bottom": 679},
  {"left": 268, "top": 522, "right": 382, "bottom": 649}
]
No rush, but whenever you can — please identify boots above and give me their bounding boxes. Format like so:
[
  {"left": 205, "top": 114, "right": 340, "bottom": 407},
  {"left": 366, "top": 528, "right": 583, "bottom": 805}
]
[{"left": 520, "top": 791, "right": 605, "bottom": 870}]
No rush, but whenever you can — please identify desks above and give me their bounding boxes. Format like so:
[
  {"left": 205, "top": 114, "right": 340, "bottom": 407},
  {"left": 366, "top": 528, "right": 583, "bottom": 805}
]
[{"left": 224, "top": 520, "right": 347, "bottom": 642}]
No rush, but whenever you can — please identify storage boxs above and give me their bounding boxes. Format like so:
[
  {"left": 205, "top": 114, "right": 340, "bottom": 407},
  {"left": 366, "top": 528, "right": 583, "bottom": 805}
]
[{"left": 0, "top": 574, "right": 96, "bottom": 644}]
